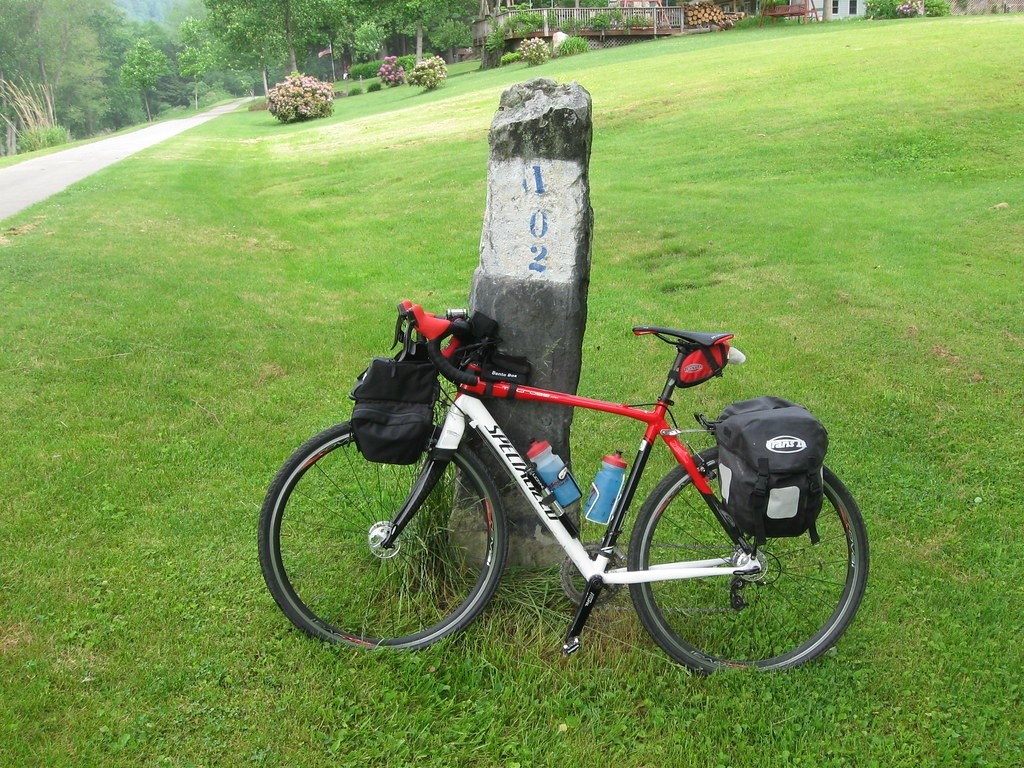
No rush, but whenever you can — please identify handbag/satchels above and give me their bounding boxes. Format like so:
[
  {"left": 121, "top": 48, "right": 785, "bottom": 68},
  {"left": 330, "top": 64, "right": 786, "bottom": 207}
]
[
  {"left": 714, "top": 395, "right": 829, "bottom": 545},
  {"left": 350, "top": 359, "right": 439, "bottom": 464}
]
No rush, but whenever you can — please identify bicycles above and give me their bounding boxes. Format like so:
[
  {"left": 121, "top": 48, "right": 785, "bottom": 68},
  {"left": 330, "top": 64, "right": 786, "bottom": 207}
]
[{"left": 258, "top": 298, "right": 868, "bottom": 682}]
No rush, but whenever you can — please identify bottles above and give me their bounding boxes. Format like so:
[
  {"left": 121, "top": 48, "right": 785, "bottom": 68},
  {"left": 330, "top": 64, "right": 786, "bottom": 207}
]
[
  {"left": 583, "top": 450, "right": 628, "bottom": 525},
  {"left": 527, "top": 437, "right": 582, "bottom": 509}
]
